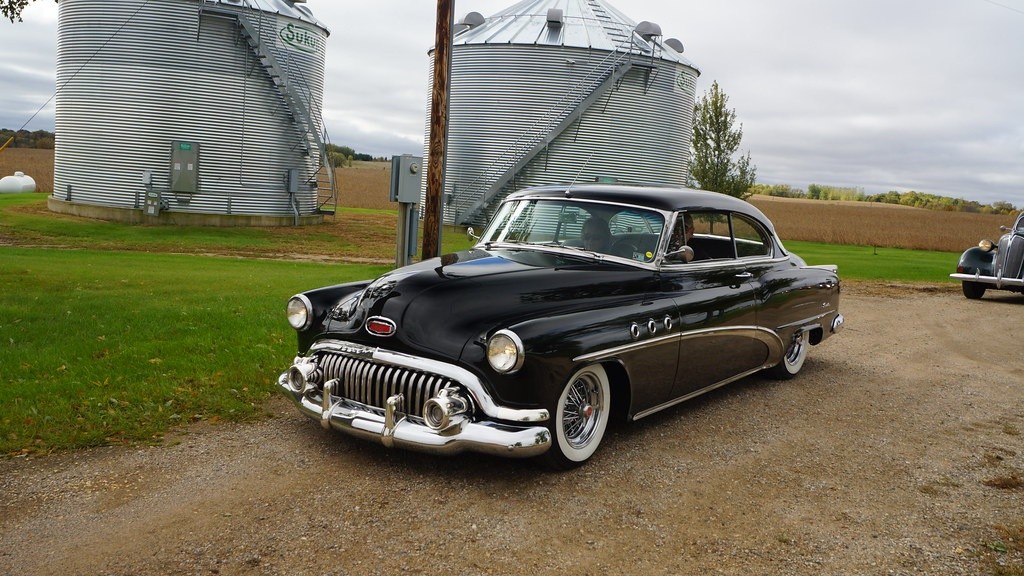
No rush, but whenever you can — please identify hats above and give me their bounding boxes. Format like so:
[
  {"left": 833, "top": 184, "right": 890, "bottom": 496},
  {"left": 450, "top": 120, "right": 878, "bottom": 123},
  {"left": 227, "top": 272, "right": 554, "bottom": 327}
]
[{"left": 684, "top": 213, "right": 693, "bottom": 228}]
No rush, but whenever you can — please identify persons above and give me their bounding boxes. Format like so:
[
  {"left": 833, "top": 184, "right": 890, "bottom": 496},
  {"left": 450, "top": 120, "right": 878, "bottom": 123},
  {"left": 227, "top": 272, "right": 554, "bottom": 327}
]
[
  {"left": 581, "top": 217, "right": 610, "bottom": 253},
  {"left": 667, "top": 215, "right": 706, "bottom": 262}
]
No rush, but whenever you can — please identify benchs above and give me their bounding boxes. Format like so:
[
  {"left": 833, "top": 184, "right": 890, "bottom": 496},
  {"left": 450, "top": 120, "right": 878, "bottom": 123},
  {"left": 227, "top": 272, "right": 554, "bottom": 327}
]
[{"left": 614, "top": 233, "right": 767, "bottom": 260}]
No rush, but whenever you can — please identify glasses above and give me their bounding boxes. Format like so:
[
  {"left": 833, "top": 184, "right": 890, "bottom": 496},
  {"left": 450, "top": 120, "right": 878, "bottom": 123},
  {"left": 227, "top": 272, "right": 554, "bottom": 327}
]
[{"left": 584, "top": 233, "right": 607, "bottom": 241}]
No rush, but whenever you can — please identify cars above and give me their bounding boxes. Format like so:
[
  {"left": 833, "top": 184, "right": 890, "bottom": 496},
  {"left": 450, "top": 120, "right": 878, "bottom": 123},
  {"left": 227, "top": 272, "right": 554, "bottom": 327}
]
[
  {"left": 273, "top": 183, "right": 846, "bottom": 463},
  {"left": 948, "top": 212, "right": 1024, "bottom": 301}
]
